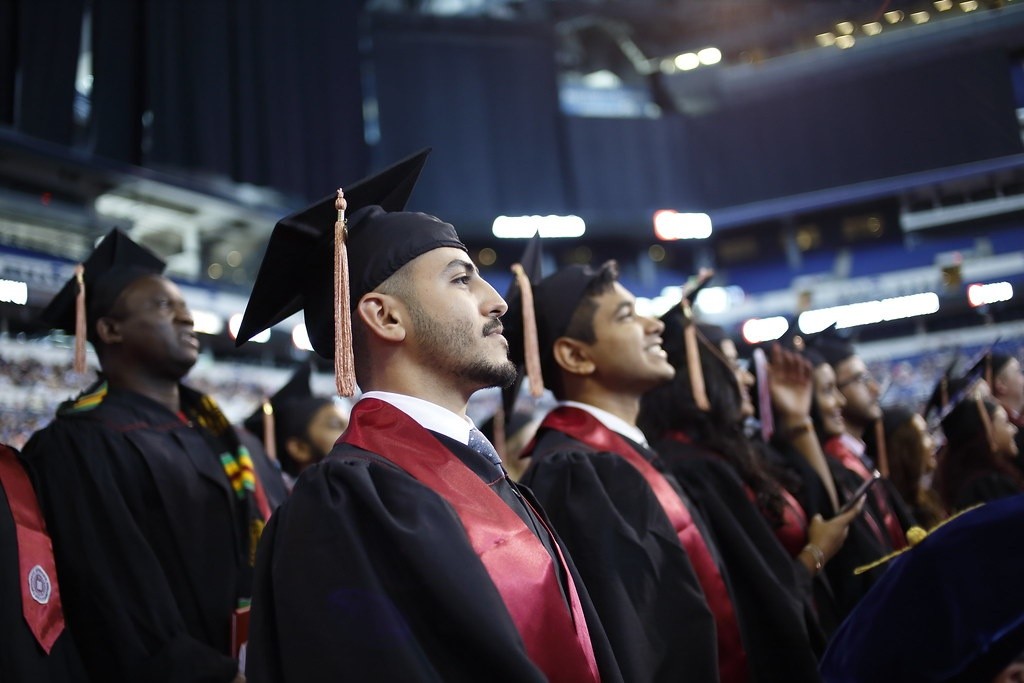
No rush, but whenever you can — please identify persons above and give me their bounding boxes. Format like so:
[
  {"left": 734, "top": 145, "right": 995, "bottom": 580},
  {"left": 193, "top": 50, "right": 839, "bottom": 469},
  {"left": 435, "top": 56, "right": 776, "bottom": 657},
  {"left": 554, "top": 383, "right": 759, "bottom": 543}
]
[
  {"left": 818, "top": 335, "right": 1024, "bottom": 683},
  {"left": 234, "top": 147, "right": 937, "bottom": 683},
  {"left": 0, "top": 229, "right": 349, "bottom": 683}
]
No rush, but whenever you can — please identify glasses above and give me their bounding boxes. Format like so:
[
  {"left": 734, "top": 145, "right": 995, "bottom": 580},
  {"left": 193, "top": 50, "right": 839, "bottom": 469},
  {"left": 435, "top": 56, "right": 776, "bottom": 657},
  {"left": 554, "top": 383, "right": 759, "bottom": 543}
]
[{"left": 839, "top": 372, "right": 873, "bottom": 390}]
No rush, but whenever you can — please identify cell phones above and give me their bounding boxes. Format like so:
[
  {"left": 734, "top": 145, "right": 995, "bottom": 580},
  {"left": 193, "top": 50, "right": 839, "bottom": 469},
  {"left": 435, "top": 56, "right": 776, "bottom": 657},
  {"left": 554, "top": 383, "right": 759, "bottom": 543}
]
[{"left": 834, "top": 470, "right": 880, "bottom": 516}]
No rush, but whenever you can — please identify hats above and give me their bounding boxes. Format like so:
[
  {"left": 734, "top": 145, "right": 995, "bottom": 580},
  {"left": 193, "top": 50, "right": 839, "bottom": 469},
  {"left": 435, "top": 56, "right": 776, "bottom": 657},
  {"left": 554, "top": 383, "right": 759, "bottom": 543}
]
[
  {"left": 235, "top": 146, "right": 468, "bottom": 396},
  {"left": 820, "top": 322, "right": 855, "bottom": 368},
  {"left": 41, "top": 228, "right": 167, "bottom": 379},
  {"left": 656, "top": 277, "right": 728, "bottom": 415},
  {"left": 927, "top": 359, "right": 1001, "bottom": 448},
  {"left": 848, "top": 405, "right": 913, "bottom": 460},
  {"left": 815, "top": 494, "right": 1024, "bottom": 683},
  {"left": 960, "top": 336, "right": 1012, "bottom": 380},
  {"left": 500, "top": 232, "right": 618, "bottom": 443},
  {"left": 742, "top": 320, "right": 815, "bottom": 443},
  {"left": 244, "top": 356, "right": 332, "bottom": 455}
]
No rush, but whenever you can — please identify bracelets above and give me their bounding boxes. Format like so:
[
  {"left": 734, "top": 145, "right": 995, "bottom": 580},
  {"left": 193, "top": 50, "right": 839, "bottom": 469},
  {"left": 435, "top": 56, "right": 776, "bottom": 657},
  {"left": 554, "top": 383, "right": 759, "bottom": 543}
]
[
  {"left": 782, "top": 421, "right": 814, "bottom": 439},
  {"left": 803, "top": 543, "right": 825, "bottom": 575}
]
